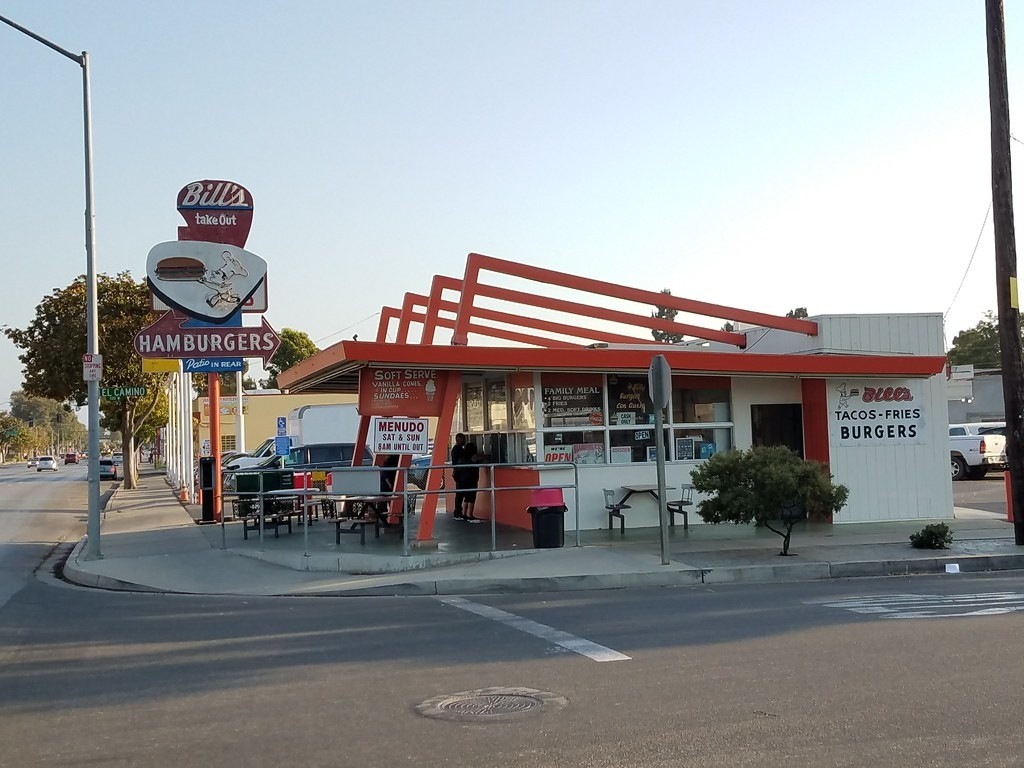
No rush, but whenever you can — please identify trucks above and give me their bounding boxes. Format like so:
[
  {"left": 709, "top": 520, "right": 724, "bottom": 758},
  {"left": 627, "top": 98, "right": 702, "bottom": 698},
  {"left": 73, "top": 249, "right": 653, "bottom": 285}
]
[{"left": 226, "top": 401, "right": 407, "bottom": 470}]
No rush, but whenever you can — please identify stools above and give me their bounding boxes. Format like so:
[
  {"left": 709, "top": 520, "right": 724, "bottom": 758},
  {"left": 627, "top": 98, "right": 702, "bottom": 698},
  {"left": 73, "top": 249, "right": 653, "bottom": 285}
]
[
  {"left": 235, "top": 498, "right": 323, "bottom": 540},
  {"left": 330, "top": 511, "right": 404, "bottom": 545},
  {"left": 667, "top": 483, "right": 694, "bottom": 530},
  {"left": 602, "top": 488, "right": 632, "bottom": 534}
]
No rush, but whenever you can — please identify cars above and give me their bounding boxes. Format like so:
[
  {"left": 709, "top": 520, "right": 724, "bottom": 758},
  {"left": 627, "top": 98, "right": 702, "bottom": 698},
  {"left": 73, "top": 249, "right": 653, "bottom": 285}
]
[
  {"left": 65, "top": 453, "right": 79, "bottom": 465},
  {"left": 59, "top": 451, "right": 89, "bottom": 461},
  {"left": 36, "top": 456, "right": 58, "bottom": 472},
  {"left": 87, "top": 458, "right": 119, "bottom": 480},
  {"left": 27, "top": 457, "right": 38, "bottom": 468},
  {"left": 112, "top": 451, "right": 124, "bottom": 464}
]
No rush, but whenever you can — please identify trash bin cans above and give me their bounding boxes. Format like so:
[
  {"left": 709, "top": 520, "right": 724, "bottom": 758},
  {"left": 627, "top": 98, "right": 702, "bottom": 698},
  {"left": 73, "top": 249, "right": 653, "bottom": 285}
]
[{"left": 526, "top": 488, "right": 568, "bottom": 548}]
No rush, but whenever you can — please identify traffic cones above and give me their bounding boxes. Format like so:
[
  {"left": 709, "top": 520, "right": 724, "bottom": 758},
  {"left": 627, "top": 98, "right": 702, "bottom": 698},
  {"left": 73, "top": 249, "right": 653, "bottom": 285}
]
[{"left": 177, "top": 483, "right": 190, "bottom": 502}]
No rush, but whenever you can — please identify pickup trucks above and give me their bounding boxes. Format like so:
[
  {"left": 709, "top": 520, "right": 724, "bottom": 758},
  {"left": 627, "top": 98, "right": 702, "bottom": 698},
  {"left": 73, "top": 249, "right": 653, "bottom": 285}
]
[{"left": 948, "top": 423, "right": 1009, "bottom": 481}]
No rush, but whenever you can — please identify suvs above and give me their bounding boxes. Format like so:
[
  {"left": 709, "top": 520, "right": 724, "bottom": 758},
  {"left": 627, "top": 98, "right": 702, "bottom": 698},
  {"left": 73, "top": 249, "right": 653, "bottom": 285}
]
[{"left": 193, "top": 441, "right": 446, "bottom": 519}]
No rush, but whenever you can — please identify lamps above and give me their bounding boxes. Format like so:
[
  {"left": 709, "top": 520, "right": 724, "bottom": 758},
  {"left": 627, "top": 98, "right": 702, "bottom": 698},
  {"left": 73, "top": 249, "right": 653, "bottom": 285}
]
[{"left": 353, "top": 334, "right": 358, "bottom": 341}]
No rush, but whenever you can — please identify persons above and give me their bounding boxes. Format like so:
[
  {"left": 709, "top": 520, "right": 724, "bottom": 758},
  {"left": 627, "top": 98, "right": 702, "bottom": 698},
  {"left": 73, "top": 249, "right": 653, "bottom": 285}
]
[{"left": 450, "top": 433, "right": 485, "bottom": 522}]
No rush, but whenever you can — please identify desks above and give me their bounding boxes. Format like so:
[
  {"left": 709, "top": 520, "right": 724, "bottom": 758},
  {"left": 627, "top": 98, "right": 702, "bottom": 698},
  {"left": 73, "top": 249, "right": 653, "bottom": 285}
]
[
  {"left": 266, "top": 488, "right": 320, "bottom": 524},
  {"left": 333, "top": 495, "right": 399, "bottom": 543},
  {"left": 609, "top": 484, "right": 688, "bottom": 512}
]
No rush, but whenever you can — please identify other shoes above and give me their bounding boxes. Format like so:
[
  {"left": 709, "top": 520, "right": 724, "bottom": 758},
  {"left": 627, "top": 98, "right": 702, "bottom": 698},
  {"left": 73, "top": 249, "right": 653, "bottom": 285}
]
[
  {"left": 453, "top": 513, "right": 465, "bottom": 520},
  {"left": 468, "top": 517, "right": 480, "bottom": 522}
]
